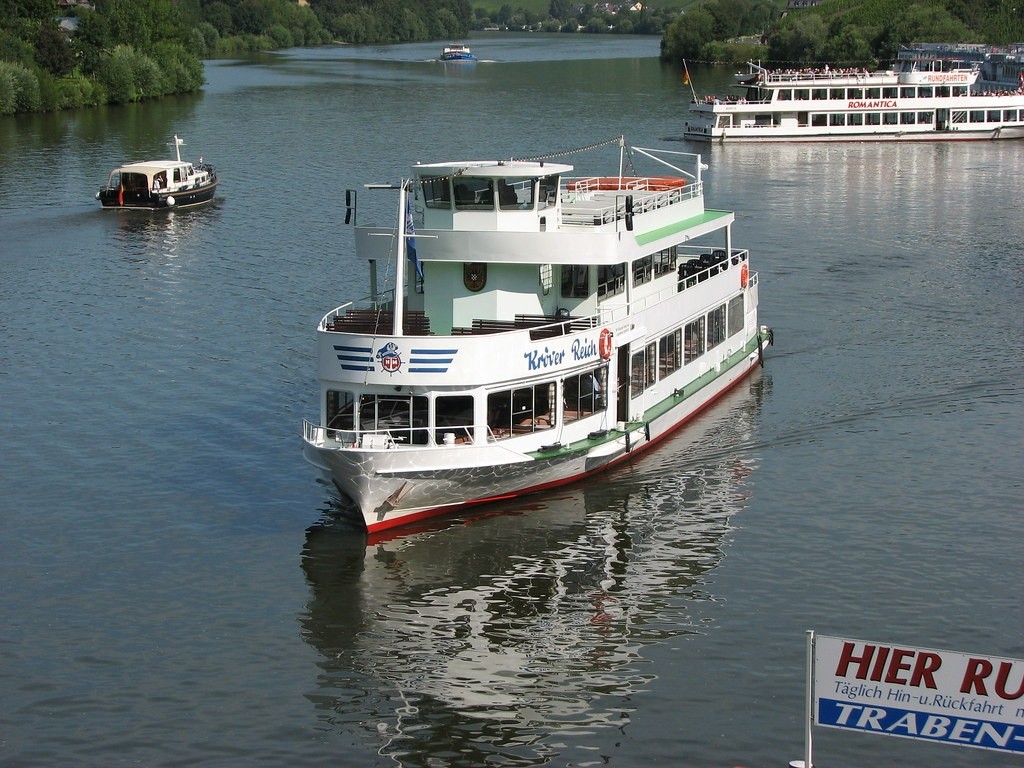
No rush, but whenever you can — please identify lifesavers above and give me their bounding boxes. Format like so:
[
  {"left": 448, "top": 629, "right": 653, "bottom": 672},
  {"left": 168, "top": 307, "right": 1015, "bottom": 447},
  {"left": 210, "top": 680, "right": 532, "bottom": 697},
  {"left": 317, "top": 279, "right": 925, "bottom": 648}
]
[
  {"left": 740, "top": 264, "right": 749, "bottom": 290},
  {"left": 598, "top": 329, "right": 612, "bottom": 360}
]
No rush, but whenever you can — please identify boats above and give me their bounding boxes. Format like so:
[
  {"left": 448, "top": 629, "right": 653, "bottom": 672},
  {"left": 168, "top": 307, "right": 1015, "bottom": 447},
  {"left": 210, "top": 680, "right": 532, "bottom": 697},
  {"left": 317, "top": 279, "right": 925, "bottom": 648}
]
[
  {"left": 95, "top": 134, "right": 220, "bottom": 211},
  {"left": 440, "top": 43, "right": 479, "bottom": 62},
  {"left": 681, "top": 59, "right": 1024, "bottom": 142},
  {"left": 301, "top": 134, "right": 775, "bottom": 535},
  {"left": 889, "top": 42, "right": 1024, "bottom": 92}
]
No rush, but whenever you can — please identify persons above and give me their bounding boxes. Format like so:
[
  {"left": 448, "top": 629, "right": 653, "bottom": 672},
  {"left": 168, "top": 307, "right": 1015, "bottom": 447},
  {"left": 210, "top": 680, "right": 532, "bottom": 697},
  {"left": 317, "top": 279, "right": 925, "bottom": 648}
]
[
  {"left": 772, "top": 64, "right": 874, "bottom": 78},
  {"left": 701, "top": 93, "right": 746, "bottom": 106},
  {"left": 972, "top": 87, "right": 1022, "bottom": 97},
  {"left": 157, "top": 174, "right": 163, "bottom": 189}
]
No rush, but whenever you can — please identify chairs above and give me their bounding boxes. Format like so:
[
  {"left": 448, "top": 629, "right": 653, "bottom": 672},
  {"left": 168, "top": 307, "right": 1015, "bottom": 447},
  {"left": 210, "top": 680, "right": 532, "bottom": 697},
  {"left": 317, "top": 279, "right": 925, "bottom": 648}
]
[{"left": 679, "top": 250, "right": 745, "bottom": 283}]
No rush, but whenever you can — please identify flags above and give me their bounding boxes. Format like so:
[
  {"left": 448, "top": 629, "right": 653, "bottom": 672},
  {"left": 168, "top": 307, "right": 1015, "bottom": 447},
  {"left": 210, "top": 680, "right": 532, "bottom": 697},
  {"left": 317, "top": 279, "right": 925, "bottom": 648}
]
[{"left": 404, "top": 191, "right": 426, "bottom": 280}]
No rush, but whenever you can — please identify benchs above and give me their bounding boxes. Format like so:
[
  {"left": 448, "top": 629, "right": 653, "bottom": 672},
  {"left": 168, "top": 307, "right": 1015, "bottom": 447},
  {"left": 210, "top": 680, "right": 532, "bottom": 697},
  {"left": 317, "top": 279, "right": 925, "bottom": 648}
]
[{"left": 323, "top": 309, "right": 596, "bottom": 340}]
